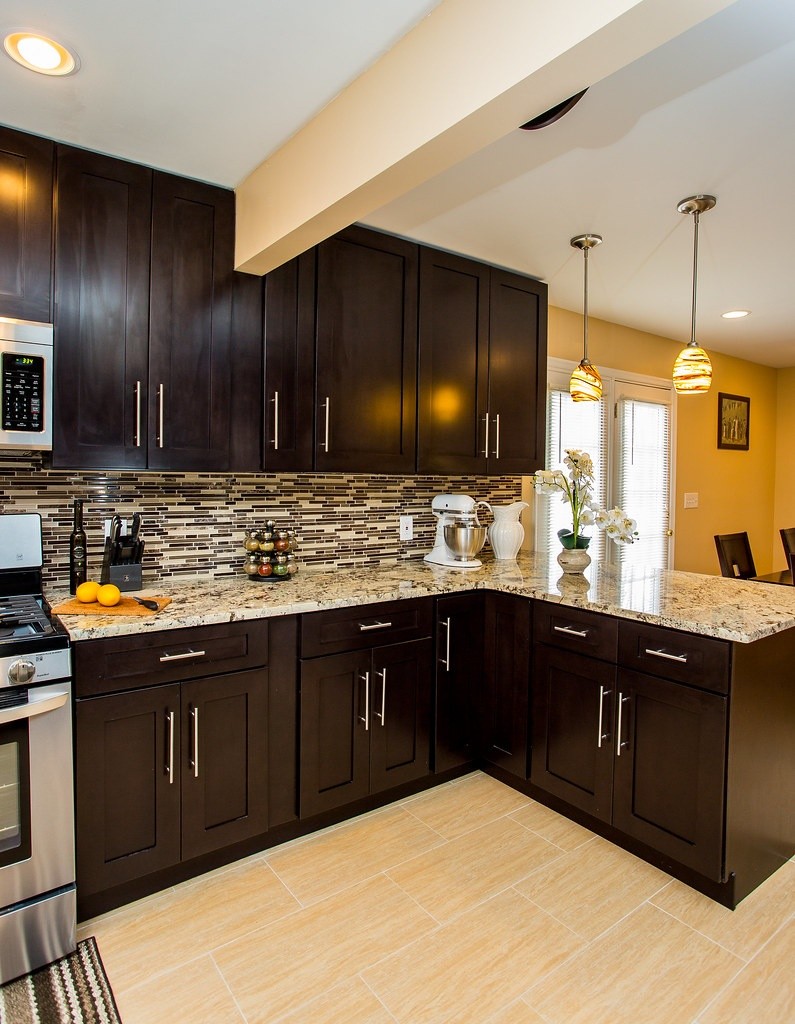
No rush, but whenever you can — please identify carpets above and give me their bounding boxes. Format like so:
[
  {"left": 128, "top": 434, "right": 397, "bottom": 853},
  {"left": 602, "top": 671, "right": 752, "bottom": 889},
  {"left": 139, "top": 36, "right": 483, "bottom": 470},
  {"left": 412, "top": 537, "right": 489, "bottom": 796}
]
[{"left": 0, "top": 936, "right": 122, "bottom": 1024}]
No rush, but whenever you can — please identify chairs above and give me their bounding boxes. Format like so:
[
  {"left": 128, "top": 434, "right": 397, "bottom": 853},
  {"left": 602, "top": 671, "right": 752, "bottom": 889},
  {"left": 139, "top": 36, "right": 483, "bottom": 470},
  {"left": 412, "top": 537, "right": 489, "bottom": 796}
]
[
  {"left": 779, "top": 527, "right": 795, "bottom": 569},
  {"left": 714, "top": 531, "right": 757, "bottom": 580}
]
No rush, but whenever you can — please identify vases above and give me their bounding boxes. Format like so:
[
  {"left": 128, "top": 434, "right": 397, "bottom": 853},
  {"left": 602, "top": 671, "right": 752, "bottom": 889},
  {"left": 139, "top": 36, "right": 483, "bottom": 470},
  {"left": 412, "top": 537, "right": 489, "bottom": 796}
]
[{"left": 557, "top": 548, "right": 591, "bottom": 574}]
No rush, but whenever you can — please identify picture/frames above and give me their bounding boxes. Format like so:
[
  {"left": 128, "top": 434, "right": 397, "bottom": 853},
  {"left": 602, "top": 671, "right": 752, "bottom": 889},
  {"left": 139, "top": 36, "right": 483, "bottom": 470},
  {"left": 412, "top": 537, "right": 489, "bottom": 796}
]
[{"left": 717, "top": 392, "right": 750, "bottom": 451}]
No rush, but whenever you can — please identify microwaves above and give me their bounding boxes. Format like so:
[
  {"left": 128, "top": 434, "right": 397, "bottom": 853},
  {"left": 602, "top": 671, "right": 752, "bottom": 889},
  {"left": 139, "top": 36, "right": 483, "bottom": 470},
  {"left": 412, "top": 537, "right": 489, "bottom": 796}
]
[{"left": 0, "top": 316, "right": 53, "bottom": 450}]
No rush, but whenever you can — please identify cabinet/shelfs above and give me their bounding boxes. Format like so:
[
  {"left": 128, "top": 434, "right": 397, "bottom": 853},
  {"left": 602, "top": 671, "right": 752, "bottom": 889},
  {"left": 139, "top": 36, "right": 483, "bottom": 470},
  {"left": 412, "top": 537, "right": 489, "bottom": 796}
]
[
  {"left": 65, "top": 590, "right": 477, "bottom": 924},
  {"left": 478, "top": 590, "right": 795, "bottom": 911},
  {"left": 0, "top": 124, "right": 548, "bottom": 480}
]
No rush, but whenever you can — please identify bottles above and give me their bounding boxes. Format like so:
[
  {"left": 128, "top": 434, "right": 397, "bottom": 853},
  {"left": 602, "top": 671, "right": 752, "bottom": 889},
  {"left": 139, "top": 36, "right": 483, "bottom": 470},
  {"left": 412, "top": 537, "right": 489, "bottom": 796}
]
[{"left": 70, "top": 500, "right": 87, "bottom": 595}]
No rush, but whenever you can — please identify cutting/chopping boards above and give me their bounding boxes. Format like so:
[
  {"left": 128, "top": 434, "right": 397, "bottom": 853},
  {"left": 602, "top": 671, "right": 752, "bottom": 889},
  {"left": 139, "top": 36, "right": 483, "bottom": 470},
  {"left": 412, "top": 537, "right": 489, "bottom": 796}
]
[{"left": 51, "top": 597, "right": 172, "bottom": 616}]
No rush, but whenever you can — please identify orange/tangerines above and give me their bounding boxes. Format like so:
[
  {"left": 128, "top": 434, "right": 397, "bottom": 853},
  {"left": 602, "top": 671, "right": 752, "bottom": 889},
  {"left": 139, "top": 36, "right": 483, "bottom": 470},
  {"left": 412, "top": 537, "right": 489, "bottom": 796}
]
[
  {"left": 76, "top": 582, "right": 100, "bottom": 602},
  {"left": 97, "top": 584, "right": 120, "bottom": 606}
]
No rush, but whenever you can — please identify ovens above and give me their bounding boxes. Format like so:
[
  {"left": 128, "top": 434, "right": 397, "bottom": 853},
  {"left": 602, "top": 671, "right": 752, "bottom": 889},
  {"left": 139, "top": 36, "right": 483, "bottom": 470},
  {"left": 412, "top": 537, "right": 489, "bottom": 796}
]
[{"left": 0, "top": 680, "right": 77, "bottom": 987}]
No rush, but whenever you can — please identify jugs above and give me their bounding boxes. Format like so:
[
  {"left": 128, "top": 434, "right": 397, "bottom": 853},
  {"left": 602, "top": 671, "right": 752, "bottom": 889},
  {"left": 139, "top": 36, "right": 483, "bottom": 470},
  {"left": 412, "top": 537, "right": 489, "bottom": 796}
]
[{"left": 474, "top": 501, "right": 529, "bottom": 559}]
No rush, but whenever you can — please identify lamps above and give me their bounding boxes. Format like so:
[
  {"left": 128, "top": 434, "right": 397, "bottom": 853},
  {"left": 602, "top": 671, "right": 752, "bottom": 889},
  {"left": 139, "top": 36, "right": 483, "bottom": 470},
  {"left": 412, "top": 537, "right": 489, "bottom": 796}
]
[
  {"left": 0, "top": 27, "right": 81, "bottom": 77},
  {"left": 569, "top": 234, "right": 604, "bottom": 401},
  {"left": 672, "top": 194, "right": 716, "bottom": 395}
]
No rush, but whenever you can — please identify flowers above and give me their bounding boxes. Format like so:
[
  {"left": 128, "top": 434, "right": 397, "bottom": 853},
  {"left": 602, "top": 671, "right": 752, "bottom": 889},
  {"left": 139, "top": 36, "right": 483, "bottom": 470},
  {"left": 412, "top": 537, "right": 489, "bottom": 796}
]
[{"left": 529, "top": 449, "right": 639, "bottom": 545}]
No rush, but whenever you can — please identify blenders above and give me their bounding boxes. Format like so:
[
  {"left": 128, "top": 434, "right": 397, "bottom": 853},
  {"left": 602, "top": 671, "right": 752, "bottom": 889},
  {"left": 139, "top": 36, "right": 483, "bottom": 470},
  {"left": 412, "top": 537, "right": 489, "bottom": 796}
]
[{"left": 423, "top": 496, "right": 482, "bottom": 567}]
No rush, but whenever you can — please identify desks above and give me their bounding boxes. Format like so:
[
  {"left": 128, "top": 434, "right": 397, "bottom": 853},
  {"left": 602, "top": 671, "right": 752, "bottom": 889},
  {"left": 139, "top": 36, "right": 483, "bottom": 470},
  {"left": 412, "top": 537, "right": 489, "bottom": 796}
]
[{"left": 748, "top": 569, "right": 792, "bottom": 585}]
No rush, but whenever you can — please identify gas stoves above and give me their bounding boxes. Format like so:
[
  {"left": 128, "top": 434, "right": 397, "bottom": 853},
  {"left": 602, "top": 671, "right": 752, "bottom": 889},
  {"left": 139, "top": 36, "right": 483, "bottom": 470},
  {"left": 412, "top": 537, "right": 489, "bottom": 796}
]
[{"left": 0, "top": 513, "right": 72, "bottom": 688}]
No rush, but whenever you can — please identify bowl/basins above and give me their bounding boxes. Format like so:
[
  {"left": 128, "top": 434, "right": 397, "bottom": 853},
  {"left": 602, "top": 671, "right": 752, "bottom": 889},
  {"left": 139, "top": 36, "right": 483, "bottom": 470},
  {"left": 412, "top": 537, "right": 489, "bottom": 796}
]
[{"left": 443, "top": 525, "right": 487, "bottom": 561}]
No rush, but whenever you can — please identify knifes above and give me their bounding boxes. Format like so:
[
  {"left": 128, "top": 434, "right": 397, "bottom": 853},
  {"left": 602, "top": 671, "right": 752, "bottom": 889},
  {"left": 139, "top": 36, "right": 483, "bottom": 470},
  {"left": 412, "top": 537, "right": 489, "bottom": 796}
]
[
  {"left": 133, "top": 597, "right": 157, "bottom": 610},
  {"left": 110, "top": 514, "right": 146, "bottom": 565}
]
